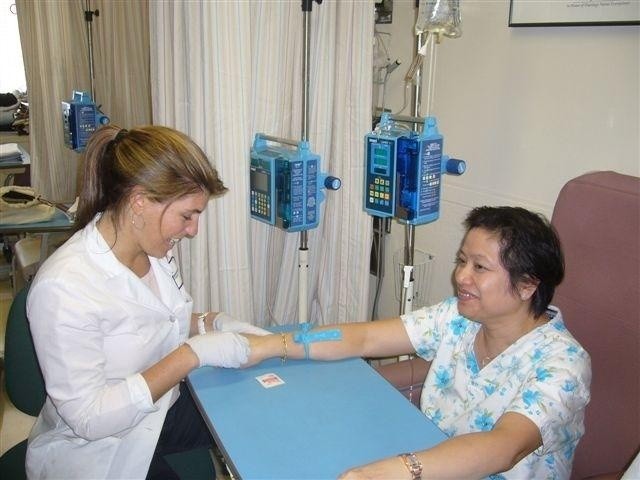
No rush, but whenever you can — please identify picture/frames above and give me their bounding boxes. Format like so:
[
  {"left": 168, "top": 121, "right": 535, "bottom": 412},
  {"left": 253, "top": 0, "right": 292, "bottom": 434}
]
[{"left": 508, "top": 0, "right": 640, "bottom": 27}]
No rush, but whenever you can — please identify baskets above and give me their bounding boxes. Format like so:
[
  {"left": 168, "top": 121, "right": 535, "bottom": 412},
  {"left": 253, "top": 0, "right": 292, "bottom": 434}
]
[{"left": 392, "top": 247, "right": 436, "bottom": 307}]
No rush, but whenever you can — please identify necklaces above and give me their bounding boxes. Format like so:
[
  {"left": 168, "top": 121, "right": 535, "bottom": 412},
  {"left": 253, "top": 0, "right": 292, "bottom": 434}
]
[{"left": 475, "top": 334, "right": 513, "bottom": 368}]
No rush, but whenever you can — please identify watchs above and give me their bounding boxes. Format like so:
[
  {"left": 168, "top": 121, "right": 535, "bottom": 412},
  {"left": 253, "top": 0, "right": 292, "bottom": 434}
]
[{"left": 398, "top": 453, "right": 422, "bottom": 480}]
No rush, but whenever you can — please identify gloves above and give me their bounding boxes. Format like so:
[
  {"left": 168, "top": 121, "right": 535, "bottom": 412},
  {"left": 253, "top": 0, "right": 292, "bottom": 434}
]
[
  {"left": 184, "top": 332, "right": 249, "bottom": 369},
  {"left": 213, "top": 312, "right": 275, "bottom": 335}
]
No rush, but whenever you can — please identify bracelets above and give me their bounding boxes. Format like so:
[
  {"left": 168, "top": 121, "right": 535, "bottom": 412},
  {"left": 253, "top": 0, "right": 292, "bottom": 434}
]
[
  {"left": 280, "top": 334, "right": 289, "bottom": 362},
  {"left": 196, "top": 310, "right": 209, "bottom": 337}
]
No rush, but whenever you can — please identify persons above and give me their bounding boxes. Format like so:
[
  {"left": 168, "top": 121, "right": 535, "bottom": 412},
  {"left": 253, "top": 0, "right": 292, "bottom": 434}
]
[
  {"left": 235, "top": 206, "right": 595, "bottom": 480},
  {"left": 24, "top": 123, "right": 271, "bottom": 480}
]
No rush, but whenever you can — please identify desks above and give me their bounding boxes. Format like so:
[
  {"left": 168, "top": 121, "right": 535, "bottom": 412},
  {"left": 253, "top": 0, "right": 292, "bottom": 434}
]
[
  {"left": 186, "top": 324, "right": 507, "bottom": 480},
  {"left": 0, "top": 203, "right": 75, "bottom": 265}
]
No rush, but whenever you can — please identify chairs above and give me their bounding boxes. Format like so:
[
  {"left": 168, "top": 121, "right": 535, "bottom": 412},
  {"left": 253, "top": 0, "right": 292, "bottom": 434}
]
[
  {"left": 0, "top": 281, "right": 216, "bottom": 480},
  {"left": 375, "top": 170, "right": 640, "bottom": 480}
]
